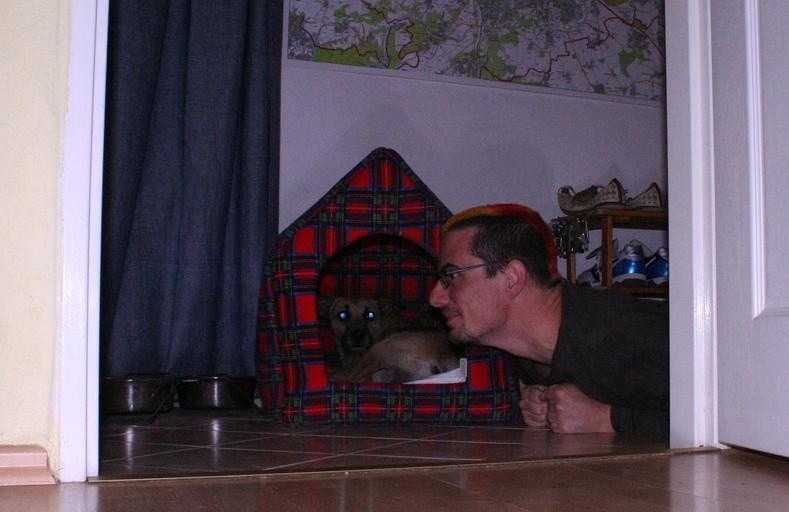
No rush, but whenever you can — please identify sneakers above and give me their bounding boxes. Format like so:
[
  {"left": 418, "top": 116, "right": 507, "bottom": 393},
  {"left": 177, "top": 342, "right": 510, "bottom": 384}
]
[
  {"left": 555, "top": 175, "right": 626, "bottom": 215},
  {"left": 576, "top": 239, "right": 647, "bottom": 287},
  {"left": 627, "top": 181, "right": 666, "bottom": 213},
  {"left": 643, "top": 243, "right": 668, "bottom": 288}
]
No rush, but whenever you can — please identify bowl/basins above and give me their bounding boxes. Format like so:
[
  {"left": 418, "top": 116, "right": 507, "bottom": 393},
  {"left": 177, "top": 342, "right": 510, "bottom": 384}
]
[
  {"left": 178, "top": 374, "right": 257, "bottom": 410},
  {"left": 104, "top": 375, "right": 174, "bottom": 415}
]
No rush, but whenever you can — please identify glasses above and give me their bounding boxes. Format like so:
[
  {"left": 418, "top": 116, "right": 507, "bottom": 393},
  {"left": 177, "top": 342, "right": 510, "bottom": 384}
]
[{"left": 439, "top": 258, "right": 507, "bottom": 291}]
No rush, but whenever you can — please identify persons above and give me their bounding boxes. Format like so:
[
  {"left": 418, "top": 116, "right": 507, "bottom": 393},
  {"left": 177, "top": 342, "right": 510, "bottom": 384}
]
[{"left": 429, "top": 204, "right": 669, "bottom": 439}]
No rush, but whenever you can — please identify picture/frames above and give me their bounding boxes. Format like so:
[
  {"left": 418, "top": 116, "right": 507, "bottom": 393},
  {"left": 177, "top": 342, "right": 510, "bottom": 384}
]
[{"left": 283, "top": 1, "right": 665, "bottom": 107}]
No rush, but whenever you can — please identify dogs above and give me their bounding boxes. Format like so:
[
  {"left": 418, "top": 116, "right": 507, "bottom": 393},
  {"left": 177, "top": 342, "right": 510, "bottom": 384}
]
[{"left": 315, "top": 291, "right": 459, "bottom": 386}]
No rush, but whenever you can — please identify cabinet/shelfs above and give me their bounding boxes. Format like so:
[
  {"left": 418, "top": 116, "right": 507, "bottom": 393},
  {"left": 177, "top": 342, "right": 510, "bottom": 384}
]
[{"left": 565, "top": 206, "right": 669, "bottom": 298}]
